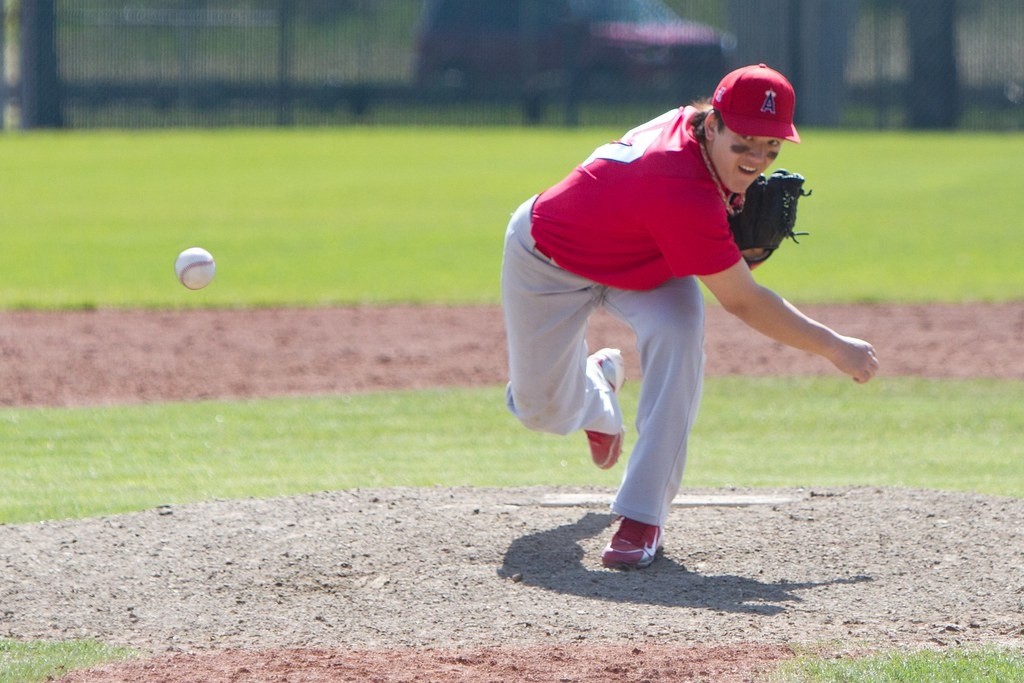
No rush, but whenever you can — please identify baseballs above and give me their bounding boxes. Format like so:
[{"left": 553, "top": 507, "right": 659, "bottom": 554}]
[{"left": 173, "top": 246, "right": 215, "bottom": 291}]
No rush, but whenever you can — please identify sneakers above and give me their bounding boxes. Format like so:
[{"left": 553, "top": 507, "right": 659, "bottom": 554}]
[
  {"left": 600, "top": 515, "right": 661, "bottom": 567},
  {"left": 583, "top": 347, "right": 628, "bottom": 470}
]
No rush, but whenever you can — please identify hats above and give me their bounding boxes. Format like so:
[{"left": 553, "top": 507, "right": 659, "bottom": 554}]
[{"left": 713, "top": 63, "right": 801, "bottom": 144}]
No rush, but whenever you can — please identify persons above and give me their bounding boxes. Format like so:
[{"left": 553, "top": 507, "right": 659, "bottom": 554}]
[{"left": 502, "top": 64, "right": 878, "bottom": 566}]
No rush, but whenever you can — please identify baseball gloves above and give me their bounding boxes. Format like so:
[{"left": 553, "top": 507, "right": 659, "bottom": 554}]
[{"left": 727, "top": 169, "right": 815, "bottom": 270}]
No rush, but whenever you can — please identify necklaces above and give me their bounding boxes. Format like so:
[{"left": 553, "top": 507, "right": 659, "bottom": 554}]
[{"left": 699, "top": 142, "right": 745, "bottom": 216}]
